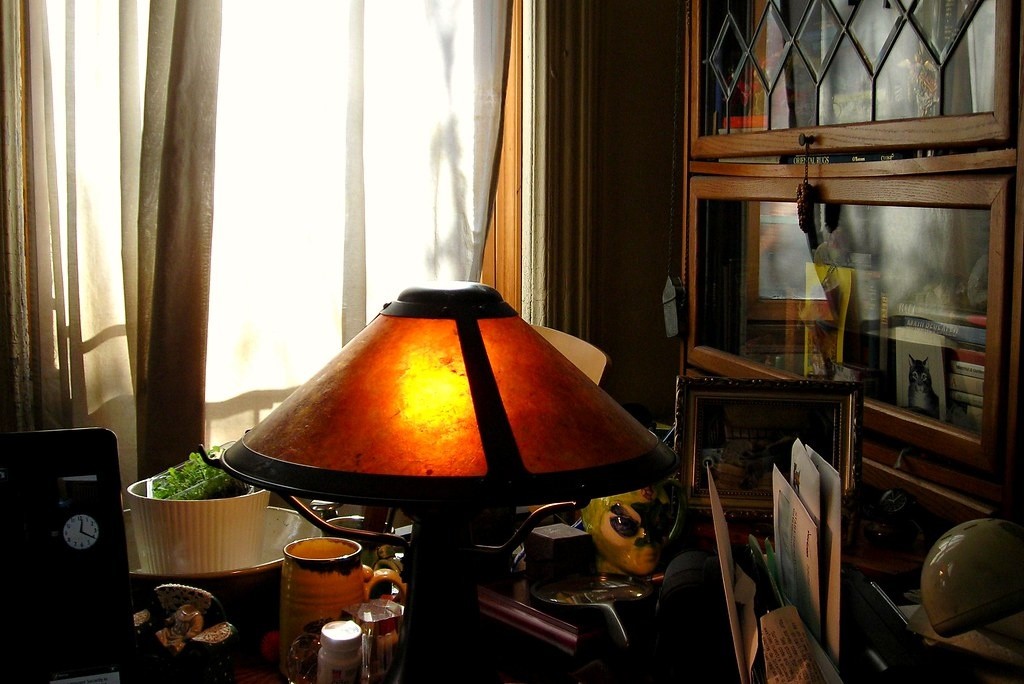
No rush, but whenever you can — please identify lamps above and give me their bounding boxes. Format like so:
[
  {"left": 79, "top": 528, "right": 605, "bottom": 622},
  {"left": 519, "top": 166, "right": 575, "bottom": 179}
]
[{"left": 221, "top": 282, "right": 684, "bottom": 684}]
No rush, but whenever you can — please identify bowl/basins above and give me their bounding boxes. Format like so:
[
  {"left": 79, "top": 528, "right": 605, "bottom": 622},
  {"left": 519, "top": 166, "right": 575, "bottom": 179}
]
[{"left": 147, "top": 435, "right": 254, "bottom": 502}]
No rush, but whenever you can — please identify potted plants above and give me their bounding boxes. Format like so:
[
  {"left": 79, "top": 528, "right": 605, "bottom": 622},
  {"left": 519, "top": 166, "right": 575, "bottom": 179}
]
[{"left": 126, "top": 452, "right": 270, "bottom": 575}]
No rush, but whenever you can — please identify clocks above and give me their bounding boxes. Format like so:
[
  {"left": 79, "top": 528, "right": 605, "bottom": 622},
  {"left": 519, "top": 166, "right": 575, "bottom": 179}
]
[{"left": 62, "top": 511, "right": 101, "bottom": 548}]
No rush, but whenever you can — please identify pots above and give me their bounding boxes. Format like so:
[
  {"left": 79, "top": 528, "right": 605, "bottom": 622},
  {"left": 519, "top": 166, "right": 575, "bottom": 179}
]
[{"left": 123, "top": 505, "right": 321, "bottom": 617}]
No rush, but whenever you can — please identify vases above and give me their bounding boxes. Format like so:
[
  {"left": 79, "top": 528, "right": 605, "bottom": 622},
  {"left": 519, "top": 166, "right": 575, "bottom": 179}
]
[{"left": 279, "top": 538, "right": 365, "bottom": 680}]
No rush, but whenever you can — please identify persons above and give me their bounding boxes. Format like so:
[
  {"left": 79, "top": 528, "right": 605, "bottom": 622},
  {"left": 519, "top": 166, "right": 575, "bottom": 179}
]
[
  {"left": 154, "top": 604, "right": 205, "bottom": 658},
  {"left": 580, "top": 482, "right": 674, "bottom": 577}
]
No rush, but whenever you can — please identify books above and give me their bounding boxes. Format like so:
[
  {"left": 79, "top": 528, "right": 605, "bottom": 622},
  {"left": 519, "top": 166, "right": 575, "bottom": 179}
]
[
  {"left": 713, "top": 0, "right": 996, "bottom": 166},
  {"left": 707, "top": 247, "right": 989, "bottom": 436}
]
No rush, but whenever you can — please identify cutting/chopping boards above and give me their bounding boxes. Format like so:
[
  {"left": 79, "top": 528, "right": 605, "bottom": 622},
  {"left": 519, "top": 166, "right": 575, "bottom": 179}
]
[{"left": 531, "top": 571, "right": 652, "bottom": 649}]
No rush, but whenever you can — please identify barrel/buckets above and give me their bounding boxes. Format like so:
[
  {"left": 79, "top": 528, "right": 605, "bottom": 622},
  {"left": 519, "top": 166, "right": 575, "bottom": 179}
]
[{"left": 127, "top": 477, "right": 271, "bottom": 570}]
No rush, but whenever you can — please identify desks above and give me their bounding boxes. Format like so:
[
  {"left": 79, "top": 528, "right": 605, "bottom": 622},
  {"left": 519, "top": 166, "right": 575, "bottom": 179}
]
[{"left": 399, "top": 525, "right": 700, "bottom": 655}]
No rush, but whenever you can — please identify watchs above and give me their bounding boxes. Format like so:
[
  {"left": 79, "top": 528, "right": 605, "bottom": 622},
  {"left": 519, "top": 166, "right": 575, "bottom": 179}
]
[{"left": 869, "top": 486, "right": 937, "bottom": 549}]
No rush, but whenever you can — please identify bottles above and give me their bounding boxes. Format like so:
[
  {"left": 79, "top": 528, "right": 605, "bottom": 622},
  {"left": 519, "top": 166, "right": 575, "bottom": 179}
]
[
  {"left": 320, "top": 620, "right": 362, "bottom": 684},
  {"left": 340, "top": 600, "right": 404, "bottom": 684},
  {"left": 280, "top": 536, "right": 366, "bottom": 678}
]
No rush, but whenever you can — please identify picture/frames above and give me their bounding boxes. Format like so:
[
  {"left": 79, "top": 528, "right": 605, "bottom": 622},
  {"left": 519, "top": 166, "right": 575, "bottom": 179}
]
[
  {"left": 686, "top": 1, "right": 1009, "bottom": 151},
  {"left": 688, "top": 171, "right": 1008, "bottom": 474},
  {"left": 674, "top": 374, "right": 862, "bottom": 521}
]
